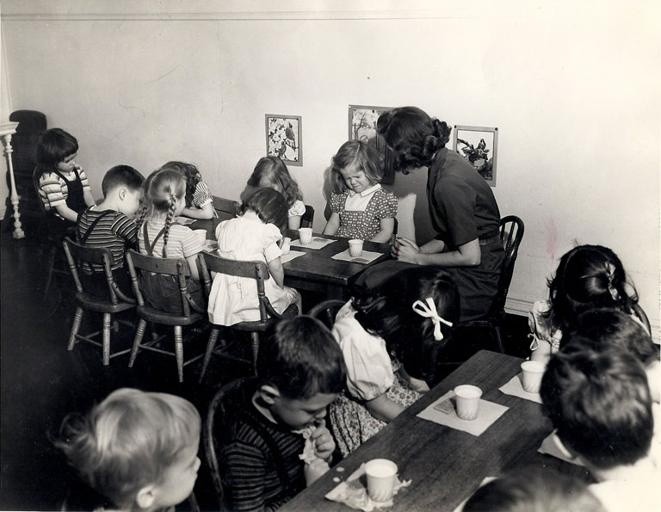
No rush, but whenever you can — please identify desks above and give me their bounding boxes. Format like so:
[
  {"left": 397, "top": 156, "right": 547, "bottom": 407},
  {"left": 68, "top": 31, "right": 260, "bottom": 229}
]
[{"left": 277, "top": 350, "right": 556, "bottom": 511}]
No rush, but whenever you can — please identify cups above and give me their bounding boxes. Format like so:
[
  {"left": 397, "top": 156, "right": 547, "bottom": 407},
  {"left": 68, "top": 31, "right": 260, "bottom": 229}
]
[
  {"left": 298, "top": 229, "right": 313, "bottom": 246},
  {"left": 281, "top": 238, "right": 291, "bottom": 255},
  {"left": 521, "top": 361, "right": 546, "bottom": 393},
  {"left": 455, "top": 384, "right": 482, "bottom": 421},
  {"left": 194, "top": 230, "right": 207, "bottom": 245},
  {"left": 348, "top": 240, "right": 363, "bottom": 257},
  {"left": 364, "top": 459, "right": 398, "bottom": 503}
]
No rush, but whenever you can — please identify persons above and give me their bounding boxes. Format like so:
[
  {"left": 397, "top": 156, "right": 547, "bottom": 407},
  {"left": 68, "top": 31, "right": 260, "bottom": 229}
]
[
  {"left": 75, "top": 164, "right": 146, "bottom": 324},
  {"left": 134, "top": 167, "right": 209, "bottom": 336},
  {"left": 239, "top": 154, "right": 307, "bottom": 230},
  {"left": 219, "top": 315, "right": 348, "bottom": 512},
  {"left": 461, "top": 467, "right": 607, "bottom": 512},
  {"left": 539, "top": 338, "right": 661, "bottom": 512},
  {"left": 161, "top": 161, "right": 219, "bottom": 220},
  {"left": 558, "top": 307, "right": 660, "bottom": 403},
  {"left": 328, "top": 265, "right": 461, "bottom": 458},
  {"left": 208, "top": 186, "right": 302, "bottom": 327},
  {"left": 527, "top": 245, "right": 652, "bottom": 363},
  {"left": 37, "top": 128, "right": 98, "bottom": 276},
  {"left": 354, "top": 106, "right": 508, "bottom": 386},
  {"left": 44, "top": 387, "right": 204, "bottom": 511},
  {"left": 322, "top": 139, "right": 399, "bottom": 244}
]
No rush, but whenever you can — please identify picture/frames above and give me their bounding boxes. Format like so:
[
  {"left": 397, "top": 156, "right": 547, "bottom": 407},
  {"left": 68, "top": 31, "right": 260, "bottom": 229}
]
[
  {"left": 348, "top": 105, "right": 393, "bottom": 143},
  {"left": 452, "top": 125, "right": 498, "bottom": 187},
  {"left": 265, "top": 114, "right": 303, "bottom": 167}
]
